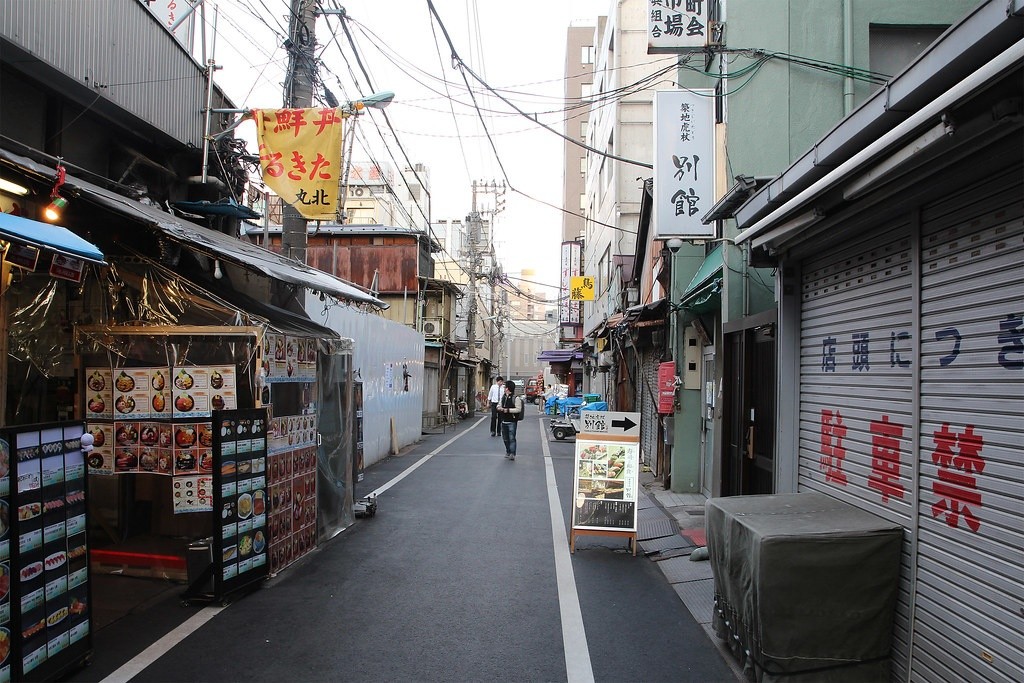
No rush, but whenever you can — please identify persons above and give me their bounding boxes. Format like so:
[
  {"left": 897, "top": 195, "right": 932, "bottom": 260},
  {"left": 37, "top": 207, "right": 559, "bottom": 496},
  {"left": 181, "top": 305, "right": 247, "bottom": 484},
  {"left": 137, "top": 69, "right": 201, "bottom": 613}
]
[
  {"left": 497, "top": 380, "right": 525, "bottom": 461},
  {"left": 538, "top": 384, "right": 553, "bottom": 404},
  {"left": 485, "top": 376, "right": 507, "bottom": 438}
]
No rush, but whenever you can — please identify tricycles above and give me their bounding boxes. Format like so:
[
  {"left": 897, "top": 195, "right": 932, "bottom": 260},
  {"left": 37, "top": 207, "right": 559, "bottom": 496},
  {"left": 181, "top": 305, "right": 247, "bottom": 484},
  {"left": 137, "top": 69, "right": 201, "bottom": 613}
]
[{"left": 550, "top": 401, "right": 607, "bottom": 441}]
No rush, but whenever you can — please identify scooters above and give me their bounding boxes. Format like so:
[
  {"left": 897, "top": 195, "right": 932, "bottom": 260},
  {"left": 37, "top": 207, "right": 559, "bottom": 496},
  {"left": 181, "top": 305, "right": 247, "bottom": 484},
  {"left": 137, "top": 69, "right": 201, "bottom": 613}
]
[{"left": 454, "top": 390, "right": 470, "bottom": 420}]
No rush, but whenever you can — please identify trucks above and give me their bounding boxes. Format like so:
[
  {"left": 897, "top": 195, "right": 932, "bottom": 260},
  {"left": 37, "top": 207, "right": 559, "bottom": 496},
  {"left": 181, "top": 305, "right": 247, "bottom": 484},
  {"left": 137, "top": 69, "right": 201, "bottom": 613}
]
[{"left": 524, "top": 378, "right": 541, "bottom": 405}]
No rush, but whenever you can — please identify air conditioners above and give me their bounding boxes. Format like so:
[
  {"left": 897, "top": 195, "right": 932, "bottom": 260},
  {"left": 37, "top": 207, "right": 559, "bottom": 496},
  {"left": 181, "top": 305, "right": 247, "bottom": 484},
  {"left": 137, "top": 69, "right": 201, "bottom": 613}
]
[{"left": 422, "top": 320, "right": 442, "bottom": 338}]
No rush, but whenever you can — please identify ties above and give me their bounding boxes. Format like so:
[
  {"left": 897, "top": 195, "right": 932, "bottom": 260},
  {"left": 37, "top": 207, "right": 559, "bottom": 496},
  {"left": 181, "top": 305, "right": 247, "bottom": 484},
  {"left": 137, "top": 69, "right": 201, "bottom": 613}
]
[{"left": 497, "top": 384, "right": 500, "bottom": 403}]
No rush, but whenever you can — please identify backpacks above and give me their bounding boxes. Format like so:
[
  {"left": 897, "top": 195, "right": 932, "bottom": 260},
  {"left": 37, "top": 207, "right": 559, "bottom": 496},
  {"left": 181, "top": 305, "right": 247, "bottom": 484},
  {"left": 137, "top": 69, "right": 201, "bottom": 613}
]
[{"left": 503, "top": 394, "right": 524, "bottom": 421}]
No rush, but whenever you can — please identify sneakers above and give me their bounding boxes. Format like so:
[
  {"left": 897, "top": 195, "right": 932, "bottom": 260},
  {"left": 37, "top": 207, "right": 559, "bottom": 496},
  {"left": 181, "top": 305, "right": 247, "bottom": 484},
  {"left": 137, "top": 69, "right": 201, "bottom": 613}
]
[
  {"left": 504, "top": 451, "right": 510, "bottom": 458},
  {"left": 508, "top": 453, "right": 514, "bottom": 461}
]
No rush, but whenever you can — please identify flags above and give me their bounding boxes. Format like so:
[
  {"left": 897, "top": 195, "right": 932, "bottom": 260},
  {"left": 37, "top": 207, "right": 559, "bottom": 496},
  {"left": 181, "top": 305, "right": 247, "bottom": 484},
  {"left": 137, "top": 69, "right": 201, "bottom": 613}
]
[{"left": 243, "top": 107, "right": 343, "bottom": 223}]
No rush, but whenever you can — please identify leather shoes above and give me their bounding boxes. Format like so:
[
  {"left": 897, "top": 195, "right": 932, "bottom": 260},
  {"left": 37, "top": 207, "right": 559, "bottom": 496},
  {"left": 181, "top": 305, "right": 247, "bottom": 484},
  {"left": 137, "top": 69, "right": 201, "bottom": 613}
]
[
  {"left": 490, "top": 431, "right": 496, "bottom": 437},
  {"left": 497, "top": 431, "right": 501, "bottom": 437}
]
